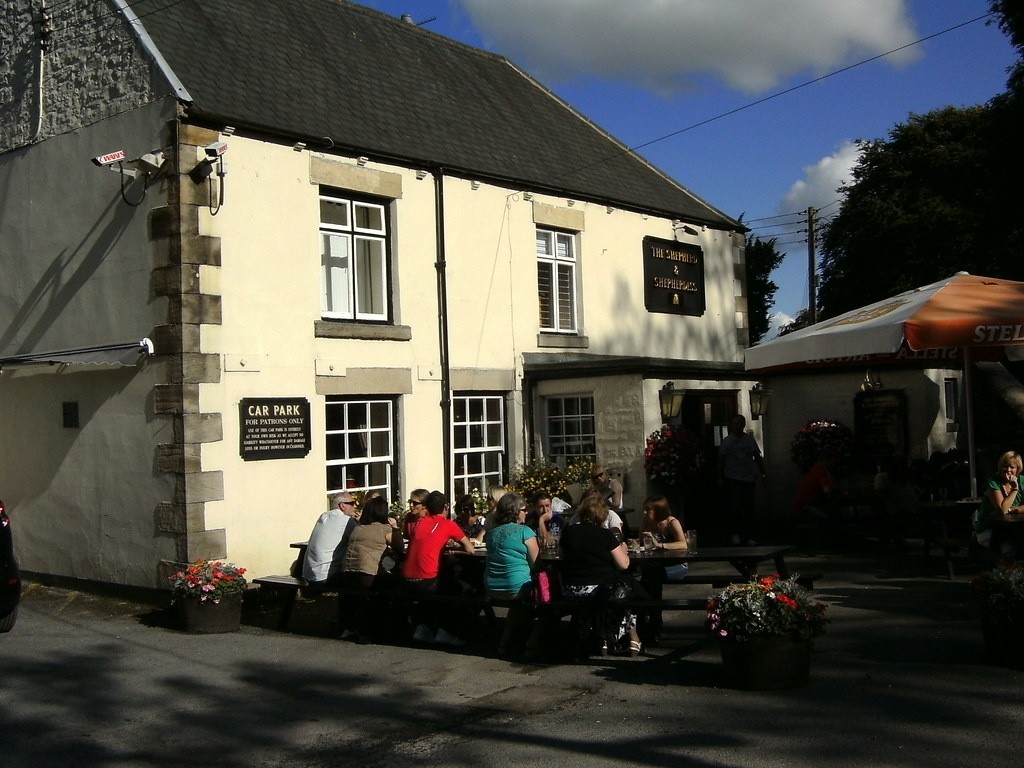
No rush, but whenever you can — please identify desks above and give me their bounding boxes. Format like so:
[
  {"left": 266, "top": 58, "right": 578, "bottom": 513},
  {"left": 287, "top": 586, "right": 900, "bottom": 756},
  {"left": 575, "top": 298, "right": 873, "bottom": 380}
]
[
  {"left": 917, "top": 493, "right": 986, "bottom": 565},
  {"left": 290, "top": 539, "right": 791, "bottom": 654},
  {"left": 987, "top": 513, "right": 1024, "bottom": 568}
]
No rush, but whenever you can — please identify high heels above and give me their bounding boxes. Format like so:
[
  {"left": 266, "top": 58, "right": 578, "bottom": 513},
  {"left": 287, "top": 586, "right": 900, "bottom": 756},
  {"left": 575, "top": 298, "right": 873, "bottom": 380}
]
[
  {"left": 629, "top": 641, "right": 642, "bottom": 657},
  {"left": 598, "top": 640, "right": 609, "bottom": 657}
]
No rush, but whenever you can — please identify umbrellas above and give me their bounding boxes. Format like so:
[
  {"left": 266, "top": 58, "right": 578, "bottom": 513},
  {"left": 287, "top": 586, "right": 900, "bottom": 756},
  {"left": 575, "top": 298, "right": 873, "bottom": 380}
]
[{"left": 744, "top": 271, "right": 1024, "bottom": 539}]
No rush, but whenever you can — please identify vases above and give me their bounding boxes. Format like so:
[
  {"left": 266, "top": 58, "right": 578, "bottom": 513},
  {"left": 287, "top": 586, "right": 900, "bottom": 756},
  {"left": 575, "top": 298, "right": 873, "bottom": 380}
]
[
  {"left": 980, "top": 619, "right": 1023, "bottom": 671},
  {"left": 716, "top": 633, "right": 814, "bottom": 689},
  {"left": 653, "top": 466, "right": 685, "bottom": 497},
  {"left": 166, "top": 591, "right": 240, "bottom": 635}
]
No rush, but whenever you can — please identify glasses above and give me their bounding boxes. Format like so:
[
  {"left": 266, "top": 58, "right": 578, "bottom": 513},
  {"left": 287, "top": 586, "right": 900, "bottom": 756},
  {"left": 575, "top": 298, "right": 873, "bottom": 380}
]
[
  {"left": 338, "top": 498, "right": 360, "bottom": 507},
  {"left": 518, "top": 507, "right": 529, "bottom": 513},
  {"left": 486, "top": 494, "right": 495, "bottom": 502},
  {"left": 457, "top": 507, "right": 474, "bottom": 514},
  {"left": 643, "top": 506, "right": 655, "bottom": 512},
  {"left": 593, "top": 472, "right": 604, "bottom": 480},
  {"left": 409, "top": 499, "right": 423, "bottom": 507}
]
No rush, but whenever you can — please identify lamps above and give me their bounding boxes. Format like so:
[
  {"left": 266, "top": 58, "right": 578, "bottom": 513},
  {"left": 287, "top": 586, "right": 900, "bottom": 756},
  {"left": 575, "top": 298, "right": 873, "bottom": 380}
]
[
  {"left": 675, "top": 224, "right": 698, "bottom": 237},
  {"left": 661, "top": 381, "right": 686, "bottom": 418},
  {"left": 749, "top": 383, "right": 776, "bottom": 416},
  {"left": 127, "top": 154, "right": 165, "bottom": 177}
]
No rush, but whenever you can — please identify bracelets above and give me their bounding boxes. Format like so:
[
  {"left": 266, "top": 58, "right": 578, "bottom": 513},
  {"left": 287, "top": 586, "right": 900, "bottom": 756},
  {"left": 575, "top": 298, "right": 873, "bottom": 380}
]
[
  {"left": 661, "top": 542, "right": 666, "bottom": 550},
  {"left": 1011, "top": 489, "right": 1018, "bottom": 491}
]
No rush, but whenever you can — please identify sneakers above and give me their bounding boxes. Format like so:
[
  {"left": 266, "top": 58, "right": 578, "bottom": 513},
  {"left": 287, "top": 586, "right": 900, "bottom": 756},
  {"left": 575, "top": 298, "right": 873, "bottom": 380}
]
[
  {"left": 413, "top": 625, "right": 434, "bottom": 643},
  {"left": 434, "top": 629, "right": 466, "bottom": 645}
]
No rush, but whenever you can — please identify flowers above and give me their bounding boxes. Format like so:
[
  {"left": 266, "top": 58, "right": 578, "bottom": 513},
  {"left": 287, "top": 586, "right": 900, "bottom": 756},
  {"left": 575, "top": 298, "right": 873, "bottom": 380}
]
[
  {"left": 971, "top": 561, "right": 1024, "bottom": 622},
  {"left": 790, "top": 415, "right": 848, "bottom": 469},
  {"left": 643, "top": 429, "right": 688, "bottom": 475},
  {"left": 170, "top": 561, "right": 242, "bottom": 604},
  {"left": 703, "top": 575, "right": 826, "bottom": 641}
]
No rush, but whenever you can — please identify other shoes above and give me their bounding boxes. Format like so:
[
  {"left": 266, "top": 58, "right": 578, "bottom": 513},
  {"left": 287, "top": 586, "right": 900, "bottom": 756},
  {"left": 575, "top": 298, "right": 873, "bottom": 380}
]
[
  {"left": 747, "top": 540, "right": 757, "bottom": 546},
  {"left": 643, "top": 633, "right": 663, "bottom": 649},
  {"left": 496, "top": 642, "right": 513, "bottom": 654},
  {"left": 731, "top": 534, "right": 740, "bottom": 544},
  {"left": 525, "top": 642, "right": 541, "bottom": 657}
]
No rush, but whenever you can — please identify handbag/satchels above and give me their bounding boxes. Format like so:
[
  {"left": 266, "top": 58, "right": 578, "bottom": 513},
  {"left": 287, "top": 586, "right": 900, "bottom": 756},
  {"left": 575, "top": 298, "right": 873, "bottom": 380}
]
[{"left": 531, "top": 570, "right": 551, "bottom": 603}]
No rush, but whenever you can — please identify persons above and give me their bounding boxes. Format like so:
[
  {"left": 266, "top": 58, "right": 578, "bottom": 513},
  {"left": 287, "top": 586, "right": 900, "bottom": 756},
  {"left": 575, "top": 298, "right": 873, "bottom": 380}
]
[
  {"left": 524, "top": 489, "right": 564, "bottom": 544},
  {"left": 559, "top": 495, "right": 647, "bottom": 656},
  {"left": 483, "top": 485, "right": 514, "bottom": 532},
  {"left": 401, "top": 488, "right": 431, "bottom": 540},
  {"left": 975, "top": 451, "right": 1024, "bottom": 557},
  {"left": 303, "top": 492, "right": 359, "bottom": 620},
  {"left": 627, "top": 494, "right": 689, "bottom": 635},
  {"left": 590, "top": 465, "right": 623, "bottom": 509},
  {"left": 718, "top": 416, "right": 767, "bottom": 547},
  {"left": 341, "top": 498, "right": 403, "bottom": 636},
  {"left": 400, "top": 491, "right": 476, "bottom": 643},
  {"left": 794, "top": 452, "right": 833, "bottom": 527},
  {"left": 352, "top": 491, "right": 399, "bottom": 528},
  {"left": 483, "top": 493, "right": 552, "bottom": 654},
  {"left": 570, "top": 489, "right": 623, "bottom": 540},
  {"left": 451, "top": 494, "right": 486, "bottom": 544}
]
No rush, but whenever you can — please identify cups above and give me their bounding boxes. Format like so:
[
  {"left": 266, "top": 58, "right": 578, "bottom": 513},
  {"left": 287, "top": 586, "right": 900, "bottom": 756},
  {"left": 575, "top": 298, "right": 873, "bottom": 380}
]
[
  {"left": 687, "top": 530, "right": 697, "bottom": 554},
  {"left": 644, "top": 538, "right": 653, "bottom": 555},
  {"left": 539, "top": 533, "right": 560, "bottom": 556},
  {"left": 685, "top": 533, "right": 696, "bottom": 544}
]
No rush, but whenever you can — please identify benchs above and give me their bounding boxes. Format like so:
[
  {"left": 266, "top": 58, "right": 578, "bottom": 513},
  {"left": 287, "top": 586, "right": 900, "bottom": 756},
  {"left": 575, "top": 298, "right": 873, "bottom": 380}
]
[{"left": 253, "top": 566, "right": 822, "bottom": 604}]
